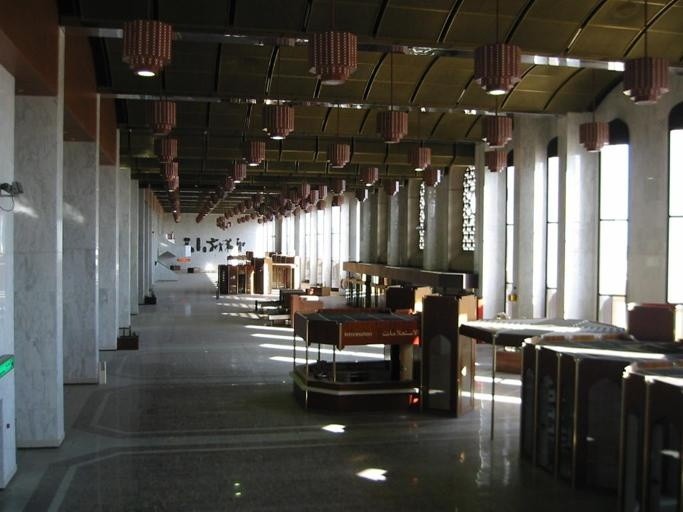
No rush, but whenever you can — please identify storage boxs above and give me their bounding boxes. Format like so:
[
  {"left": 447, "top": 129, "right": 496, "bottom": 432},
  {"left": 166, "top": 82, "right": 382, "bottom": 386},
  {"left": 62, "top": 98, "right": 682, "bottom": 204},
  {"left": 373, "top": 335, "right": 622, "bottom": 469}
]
[
  {"left": 494, "top": 345, "right": 522, "bottom": 374},
  {"left": 621, "top": 298, "right": 683, "bottom": 348}
]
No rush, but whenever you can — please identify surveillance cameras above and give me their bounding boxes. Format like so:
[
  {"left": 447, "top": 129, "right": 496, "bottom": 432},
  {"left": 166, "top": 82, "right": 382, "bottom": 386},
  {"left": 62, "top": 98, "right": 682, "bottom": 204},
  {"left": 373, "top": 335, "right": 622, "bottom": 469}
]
[{"left": 0, "top": 181, "right": 25, "bottom": 195}]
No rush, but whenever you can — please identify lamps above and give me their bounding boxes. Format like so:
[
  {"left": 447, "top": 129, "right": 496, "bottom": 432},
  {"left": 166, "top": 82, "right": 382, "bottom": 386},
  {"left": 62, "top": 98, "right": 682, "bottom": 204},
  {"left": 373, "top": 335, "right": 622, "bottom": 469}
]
[
  {"left": 413, "top": 112, "right": 441, "bottom": 187},
  {"left": 474, "top": 2, "right": 522, "bottom": 96},
  {"left": 379, "top": 49, "right": 408, "bottom": 143},
  {"left": 262, "top": 44, "right": 295, "bottom": 140},
  {"left": 356, "top": 165, "right": 379, "bottom": 203},
  {"left": 216, "top": 177, "right": 346, "bottom": 230},
  {"left": 384, "top": 165, "right": 400, "bottom": 195},
  {"left": 122, "top": 3, "right": 171, "bottom": 76},
  {"left": 482, "top": 97, "right": 513, "bottom": 172},
  {"left": 195, "top": 159, "right": 246, "bottom": 224},
  {"left": 580, "top": 68, "right": 608, "bottom": 152},
  {"left": 153, "top": 82, "right": 181, "bottom": 224},
  {"left": 624, "top": 2, "right": 671, "bottom": 106},
  {"left": 244, "top": 104, "right": 265, "bottom": 167},
  {"left": 328, "top": 103, "right": 350, "bottom": 168},
  {"left": 310, "top": 2, "right": 357, "bottom": 85}
]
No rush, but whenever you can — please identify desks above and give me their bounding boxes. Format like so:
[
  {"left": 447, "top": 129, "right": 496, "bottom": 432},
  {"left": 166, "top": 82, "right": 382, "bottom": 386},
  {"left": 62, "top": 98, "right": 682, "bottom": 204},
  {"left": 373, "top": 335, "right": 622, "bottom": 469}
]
[
  {"left": 292, "top": 301, "right": 423, "bottom": 383},
  {"left": 516, "top": 335, "right": 683, "bottom": 488},
  {"left": 613, "top": 356, "right": 683, "bottom": 511},
  {"left": 456, "top": 318, "right": 624, "bottom": 441}
]
